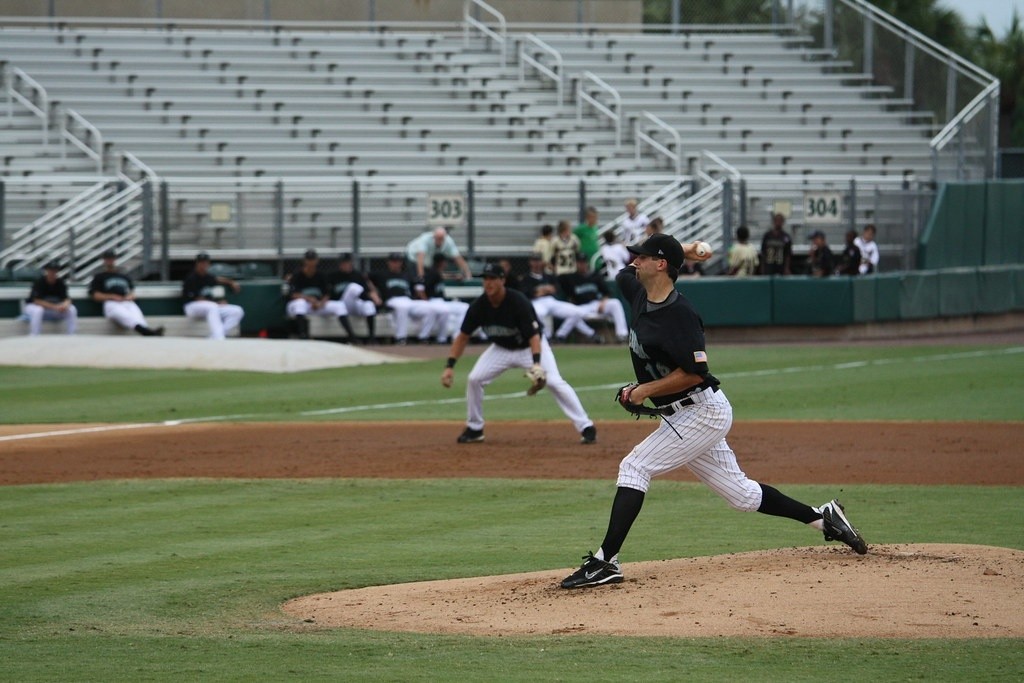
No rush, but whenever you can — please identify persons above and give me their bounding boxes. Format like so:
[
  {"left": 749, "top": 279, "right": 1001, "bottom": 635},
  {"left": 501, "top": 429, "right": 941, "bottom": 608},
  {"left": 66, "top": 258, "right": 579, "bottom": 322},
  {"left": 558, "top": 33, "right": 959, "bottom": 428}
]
[
  {"left": 87, "top": 248, "right": 166, "bottom": 337},
  {"left": 441, "top": 263, "right": 598, "bottom": 445},
  {"left": 283, "top": 251, "right": 384, "bottom": 347},
  {"left": 499, "top": 199, "right": 665, "bottom": 347},
  {"left": 183, "top": 253, "right": 244, "bottom": 342},
  {"left": 562, "top": 234, "right": 866, "bottom": 590},
  {"left": 372, "top": 225, "right": 471, "bottom": 345},
  {"left": 22, "top": 261, "right": 77, "bottom": 336},
  {"left": 807, "top": 224, "right": 880, "bottom": 277},
  {"left": 728, "top": 213, "right": 793, "bottom": 277}
]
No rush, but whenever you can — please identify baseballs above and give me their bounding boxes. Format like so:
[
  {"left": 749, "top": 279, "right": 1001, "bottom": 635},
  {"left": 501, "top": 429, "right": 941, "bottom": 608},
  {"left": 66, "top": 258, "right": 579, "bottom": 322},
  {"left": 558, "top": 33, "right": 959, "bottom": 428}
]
[{"left": 696, "top": 242, "right": 712, "bottom": 257}]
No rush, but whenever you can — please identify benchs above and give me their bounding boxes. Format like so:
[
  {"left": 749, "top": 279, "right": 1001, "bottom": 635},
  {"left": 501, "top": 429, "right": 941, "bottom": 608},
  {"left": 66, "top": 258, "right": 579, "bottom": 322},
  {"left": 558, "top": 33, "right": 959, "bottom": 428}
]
[
  {"left": 306, "top": 286, "right": 518, "bottom": 339},
  {"left": 0, "top": 15, "right": 986, "bottom": 254},
  {"left": 1, "top": 284, "right": 235, "bottom": 336}
]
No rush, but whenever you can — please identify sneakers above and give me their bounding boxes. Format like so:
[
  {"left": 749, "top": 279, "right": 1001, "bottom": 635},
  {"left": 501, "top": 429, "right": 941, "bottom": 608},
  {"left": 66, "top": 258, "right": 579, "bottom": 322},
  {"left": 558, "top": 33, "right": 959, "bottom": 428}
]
[
  {"left": 559, "top": 549, "right": 625, "bottom": 590},
  {"left": 817, "top": 499, "right": 867, "bottom": 556},
  {"left": 580, "top": 425, "right": 598, "bottom": 444},
  {"left": 456, "top": 426, "right": 486, "bottom": 442}
]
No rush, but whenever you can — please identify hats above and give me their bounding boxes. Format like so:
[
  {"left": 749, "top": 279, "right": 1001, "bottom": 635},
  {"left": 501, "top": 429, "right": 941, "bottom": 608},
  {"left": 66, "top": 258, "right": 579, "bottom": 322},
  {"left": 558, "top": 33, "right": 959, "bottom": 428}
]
[
  {"left": 530, "top": 252, "right": 544, "bottom": 262},
  {"left": 771, "top": 211, "right": 786, "bottom": 226},
  {"left": 473, "top": 261, "right": 507, "bottom": 279},
  {"left": 103, "top": 250, "right": 118, "bottom": 259},
  {"left": 625, "top": 232, "right": 684, "bottom": 269},
  {"left": 44, "top": 260, "right": 61, "bottom": 270},
  {"left": 808, "top": 231, "right": 825, "bottom": 239},
  {"left": 195, "top": 254, "right": 211, "bottom": 262},
  {"left": 305, "top": 251, "right": 318, "bottom": 261},
  {"left": 337, "top": 253, "right": 352, "bottom": 262},
  {"left": 388, "top": 253, "right": 403, "bottom": 263},
  {"left": 576, "top": 252, "right": 588, "bottom": 262}
]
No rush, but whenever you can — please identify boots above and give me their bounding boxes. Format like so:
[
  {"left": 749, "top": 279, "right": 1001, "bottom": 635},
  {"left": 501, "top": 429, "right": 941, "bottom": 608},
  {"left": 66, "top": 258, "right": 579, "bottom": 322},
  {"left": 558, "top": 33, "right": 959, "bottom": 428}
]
[
  {"left": 134, "top": 324, "right": 164, "bottom": 338},
  {"left": 285, "top": 319, "right": 295, "bottom": 338},
  {"left": 337, "top": 315, "right": 356, "bottom": 345},
  {"left": 367, "top": 315, "right": 379, "bottom": 345},
  {"left": 295, "top": 314, "right": 309, "bottom": 338}
]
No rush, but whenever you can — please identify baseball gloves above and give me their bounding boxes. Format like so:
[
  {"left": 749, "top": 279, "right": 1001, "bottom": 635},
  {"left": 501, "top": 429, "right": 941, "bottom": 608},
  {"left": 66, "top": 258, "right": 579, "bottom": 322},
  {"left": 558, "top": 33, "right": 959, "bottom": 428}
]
[
  {"left": 618, "top": 385, "right": 664, "bottom": 415},
  {"left": 523, "top": 367, "right": 547, "bottom": 395}
]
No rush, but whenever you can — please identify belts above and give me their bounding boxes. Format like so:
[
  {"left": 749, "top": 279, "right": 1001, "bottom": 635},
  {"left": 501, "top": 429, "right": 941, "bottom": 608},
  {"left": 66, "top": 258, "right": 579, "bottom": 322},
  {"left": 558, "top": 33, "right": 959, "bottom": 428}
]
[{"left": 655, "top": 384, "right": 721, "bottom": 418}]
